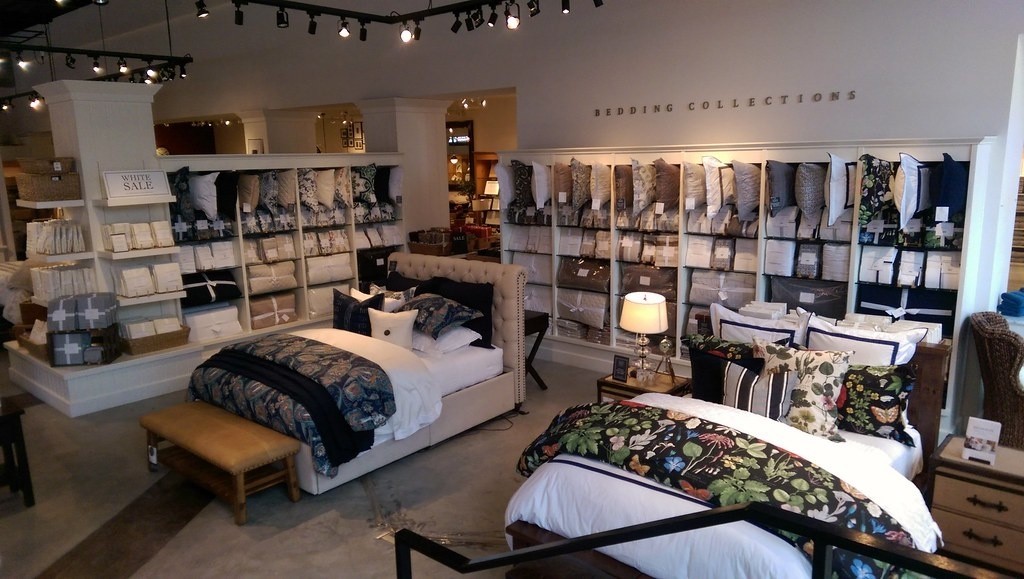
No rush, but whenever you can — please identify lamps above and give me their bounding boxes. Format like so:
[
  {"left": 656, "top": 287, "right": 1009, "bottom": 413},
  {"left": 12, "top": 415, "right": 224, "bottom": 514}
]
[
  {"left": 306, "top": 11, "right": 321, "bottom": 34},
  {"left": 451, "top": 0, "right": 520, "bottom": 33},
  {"left": 0, "top": 97, "right": 8, "bottom": 109},
  {"left": 619, "top": 292, "right": 668, "bottom": 381},
  {"left": 358, "top": 19, "right": 371, "bottom": 41},
  {"left": 460, "top": 98, "right": 487, "bottom": 109},
  {"left": 93, "top": 0, "right": 109, "bottom": 6},
  {"left": 64, "top": 52, "right": 186, "bottom": 85},
  {"left": 592, "top": 0, "right": 603, "bottom": 8},
  {"left": 412, "top": 17, "right": 424, "bottom": 40},
  {"left": 29, "top": 94, "right": 39, "bottom": 107},
  {"left": 231, "top": 0, "right": 249, "bottom": 25},
  {"left": 16, "top": 50, "right": 24, "bottom": 66},
  {"left": 526, "top": 0, "right": 540, "bottom": 17},
  {"left": 277, "top": 5, "right": 289, "bottom": 28},
  {"left": 472, "top": 199, "right": 491, "bottom": 227},
  {"left": 195, "top": 0, "right": 209, "bottom": 18},
  {"left": 399, "top": 20, "right": 412, "bottom": 41},
  {"left": 337, "top": 17, "right": 350, "bottom": 38},
  {"left": 561, "top": 0, "right": 570, "bottom": 13}
]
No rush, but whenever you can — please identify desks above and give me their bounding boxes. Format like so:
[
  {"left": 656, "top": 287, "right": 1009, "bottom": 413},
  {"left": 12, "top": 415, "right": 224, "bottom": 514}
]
[
  {"left": 525, "top": 309, "right": 550, "bottom": 392},
  {"left": 0, "top": 396, "right": 34, "bottom": 507},
  {"left": 597, "top": 365, "right": 693, "bottom": 403}
]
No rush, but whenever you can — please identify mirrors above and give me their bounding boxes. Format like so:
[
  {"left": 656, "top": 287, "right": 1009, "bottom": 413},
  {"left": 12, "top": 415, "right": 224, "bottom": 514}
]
[{"left": 445, "top": 120, "right": 475, "bottom": 191}]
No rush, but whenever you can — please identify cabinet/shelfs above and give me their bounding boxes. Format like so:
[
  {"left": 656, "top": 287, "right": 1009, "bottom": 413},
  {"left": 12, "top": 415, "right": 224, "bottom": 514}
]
[
  {"left": 92, "top": 194, "right": 187, "bottom": 307},
  {"left": 15, "top": 199, "right": 94, "bottom": 308},
  {"left": 491, "top": 134, "right": 995, "bottom": 383},
  {"left": 471, "top": 153, "right": 501, "bottom": 228},
  {"left": 154, "top": 152, "right": 408, "bottom": 359}
]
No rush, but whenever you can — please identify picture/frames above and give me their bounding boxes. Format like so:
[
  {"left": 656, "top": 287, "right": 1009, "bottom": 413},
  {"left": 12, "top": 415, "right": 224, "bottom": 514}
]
[
  {"left": 656, "top": 351, "right": 675, "bottom": 384},
  {"left": 611, "top": 354, "right": 629, "bottom": 383},
  {"left": 339, "top": 122, "right": 366, "bottom": 150}
]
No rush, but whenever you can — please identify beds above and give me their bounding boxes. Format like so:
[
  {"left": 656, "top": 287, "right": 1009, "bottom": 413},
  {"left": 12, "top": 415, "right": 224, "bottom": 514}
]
[
  {"left": 503, "top": 312, "right": 951, "bottom": 578},
  {"left": 0, "top": 261, "right": 32, "bottom": 324},
  {"left": 185, "top": 249, "right": 527, "bottom": 495}
]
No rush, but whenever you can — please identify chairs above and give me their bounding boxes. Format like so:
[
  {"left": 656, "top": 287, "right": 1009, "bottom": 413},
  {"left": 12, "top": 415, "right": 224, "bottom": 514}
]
[{"left": 970, "top": 311, "right": 1024, "bottom": 454}]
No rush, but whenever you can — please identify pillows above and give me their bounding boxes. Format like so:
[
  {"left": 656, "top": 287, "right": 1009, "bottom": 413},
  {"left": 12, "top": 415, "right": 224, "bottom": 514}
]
[
  {"left": 679, "top": 302, "right": 930, "bottom": 448},
  {"left": 510, "top": 152, "right": 967, "bottom": 230},
  {"left": 169, "top": 165, "right": 407, "bottom": 228},
  {"left": 331, "top": 270, "right": 496, "bottom": 354}
]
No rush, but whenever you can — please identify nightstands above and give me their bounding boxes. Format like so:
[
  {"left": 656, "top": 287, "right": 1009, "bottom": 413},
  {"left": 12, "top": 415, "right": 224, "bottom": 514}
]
[{"left": 921, "top": 433, "right": 1024, "bottom": 577}]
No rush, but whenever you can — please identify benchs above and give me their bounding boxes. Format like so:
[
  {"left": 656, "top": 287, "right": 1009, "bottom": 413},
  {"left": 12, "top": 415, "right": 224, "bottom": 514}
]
[{"left": 140, "top": 400, "right": 303, "bottom": 527}]
